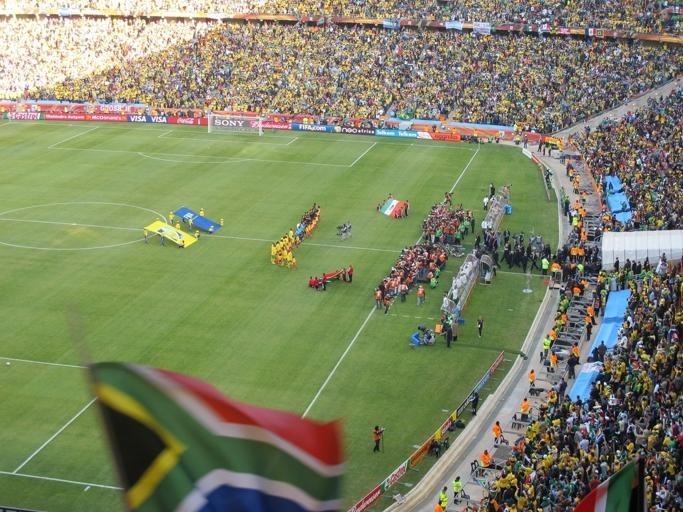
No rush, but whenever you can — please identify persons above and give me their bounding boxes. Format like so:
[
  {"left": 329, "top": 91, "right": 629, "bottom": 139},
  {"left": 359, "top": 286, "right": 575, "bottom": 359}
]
[
  {"left": 336, "top": 220, "right": 353, "bottom": 240},
  {"left": 168, "top": 211, "right": 174, "bottom": 225},
  {"left": 178, "top": 240, "right": 185, "bottom": 249},
  {"left": 142, "top": 228, "right": 148, "bottom": 242},
  {"left": 307, "top": 264, "right": 354, "bottom": 291},
  {"left": 0, "top": 0, "right": 683, "bottom": 125},
  {"left": 270, "top": 202, "right": 320, "bottom": 272},
  {"left": 159, "top": 233, "right": 164, "bottom": 245},
  {"left": 188, "top": 216, "right": 193, "bottom": 229},
  {"left": 199, "top": 208, "right": 204, "bottom": 216},
  {"left": 219, "top": 218, "right": 224, "bottom": 228},
  {"left": 194, "top": 229, "right": 200, "bottom": 240},
  {"left": 175, "top": 221, "right": 180, "bottom": 229},
  {"left": 372, "top": 126, "right": 682, "bottom": 512},
  {"left": 207, "top": 225, "right": 214, "bottom": 234}
]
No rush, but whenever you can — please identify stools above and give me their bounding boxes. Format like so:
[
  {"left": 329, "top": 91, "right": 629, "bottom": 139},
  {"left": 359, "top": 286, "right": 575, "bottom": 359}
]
[{"left": 555, "top": 269, "right": 564, "bottom": 281}]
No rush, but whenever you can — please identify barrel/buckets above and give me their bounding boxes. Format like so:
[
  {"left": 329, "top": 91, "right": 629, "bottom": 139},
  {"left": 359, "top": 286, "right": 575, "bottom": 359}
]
[{"left": 506, "top": 205, "right": 512, "bottom": 215}]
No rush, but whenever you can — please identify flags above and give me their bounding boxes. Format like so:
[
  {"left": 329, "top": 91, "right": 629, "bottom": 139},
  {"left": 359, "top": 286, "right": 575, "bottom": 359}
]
[{"left": 85, "top": 357, "right": 349, "bottom": 510}]
